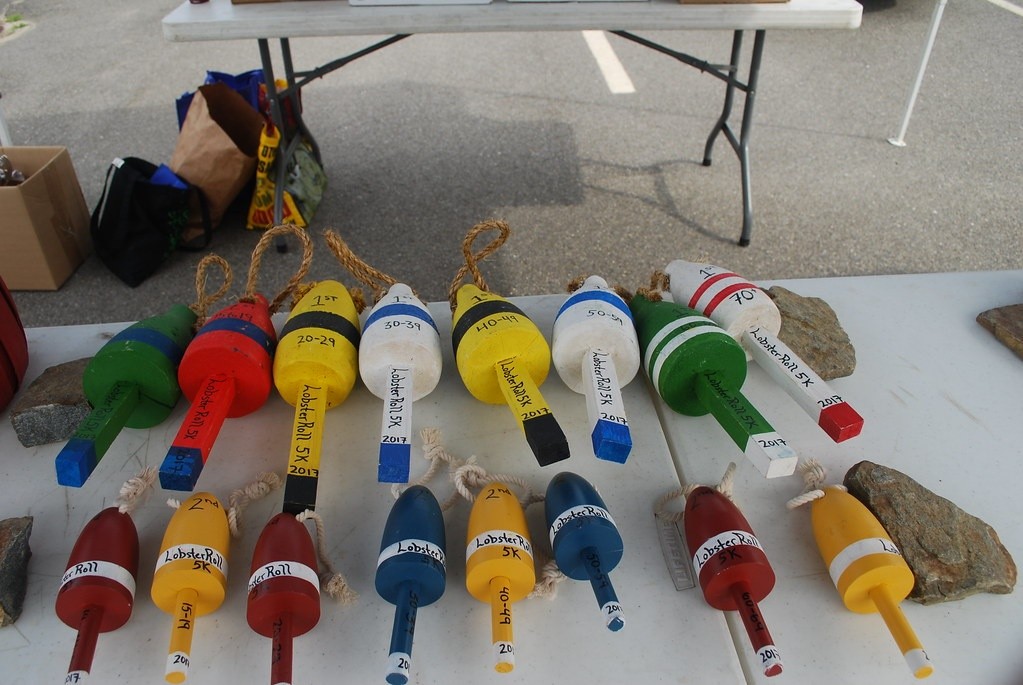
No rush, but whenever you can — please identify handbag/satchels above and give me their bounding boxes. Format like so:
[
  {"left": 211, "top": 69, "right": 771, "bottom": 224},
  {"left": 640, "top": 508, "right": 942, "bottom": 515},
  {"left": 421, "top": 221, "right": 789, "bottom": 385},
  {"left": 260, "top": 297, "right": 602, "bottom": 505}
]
[
  {"left": 175, "top": 67, "right": 265, "bottom": 134},
  {"left": 88, "top": 156, "right": 213, "bottom": 288},
  {"left": 245, "top": 77, "right": 326, "bottom": 230}
]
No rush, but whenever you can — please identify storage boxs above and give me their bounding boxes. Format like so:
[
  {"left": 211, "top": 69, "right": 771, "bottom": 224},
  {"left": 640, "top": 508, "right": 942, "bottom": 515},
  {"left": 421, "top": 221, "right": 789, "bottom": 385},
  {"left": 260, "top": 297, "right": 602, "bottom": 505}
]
[{"left": 0, "top": 147, "right": 94, "bottom": 291}]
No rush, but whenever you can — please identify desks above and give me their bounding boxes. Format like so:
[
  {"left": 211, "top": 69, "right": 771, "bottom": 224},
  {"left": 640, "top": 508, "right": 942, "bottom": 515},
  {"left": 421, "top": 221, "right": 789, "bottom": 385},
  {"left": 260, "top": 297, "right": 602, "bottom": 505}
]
[{"left": 161, "top": 0, "right": 863, "bottom": 248}]
